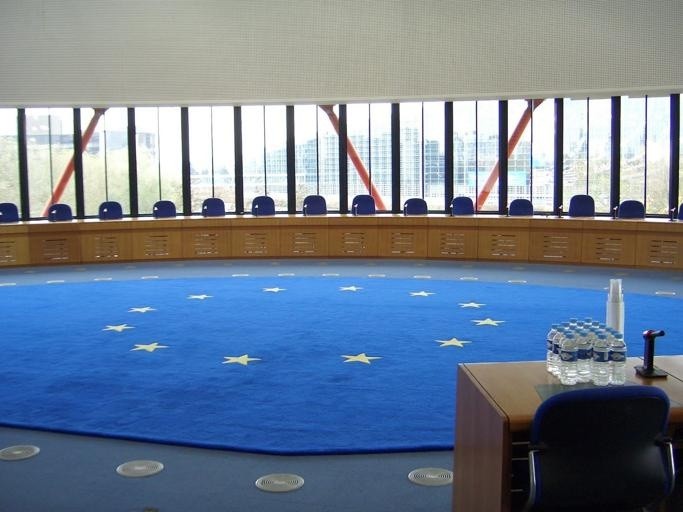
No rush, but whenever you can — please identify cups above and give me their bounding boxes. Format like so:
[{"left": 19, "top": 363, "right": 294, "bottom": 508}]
[{"left": 606, "top": 279, "right": 624, "bottom": 337}]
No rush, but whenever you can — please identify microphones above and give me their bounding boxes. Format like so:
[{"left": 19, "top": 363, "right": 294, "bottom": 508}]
[
  {"left": 634, "top": 330, "right": 668, "bottom": 378},
  {"left": 103, "top": 208, "right": 107, "bottom": 219},
  {"left": 669, "top": 207, "right": 677, "bottom": 221},
  {"left": 255, "top": 205, "right": 259, "bottom": 216},
  {"left": 557, "top": 205, "right": 563, "bottom": 218},
  {"left": 505, "top": 204, "right": 510, "bottom": 217},
  {"left": 51, "top": 210, "right": 57, "bottom": 221},
  {"left": 354, "top": 204, "right": 360, "bottom": 216},
  {"left": 154, "top": 206, "right": 160, "bottom": 219},
  {"left": 449, "top": 204, "right": 454, "bottom": 217},
  {"left": 612, "top": 206, "right": 619, "bottom": 219},
  {"left": 204, "top": 206, "right": 208, "bottom": 217},
  {"left": 404, "top": 204, "right": 408, "bottom": 216},
  {"left": 303, "top": 204, "right": 308, "bottom": 216}
]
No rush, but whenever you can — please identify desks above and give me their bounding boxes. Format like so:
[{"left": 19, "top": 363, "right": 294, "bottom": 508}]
[
  {"left": 453, "top": 353, "right": 681, "bottom": 509},
  {"left": 640, "top": 354, "right": 681, "bottom": 380}
]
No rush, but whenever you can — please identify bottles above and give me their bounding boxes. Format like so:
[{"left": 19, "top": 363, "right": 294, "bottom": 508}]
[
  {"left": 612, "top": 208, "right": 615, "bottom": 219},
  {"left": 254, "top": 205, "right": 257, "bottom": 216},
  {"left": 102, "top": 207, "right": 107, "bottom": 219},
  {"left": 203, "top": 206, "right": 207, "bottom": 217},
  {"left": 404, "top": 205, "right": 406, "bottom": 217},
  {"left": 449, "top": 204, "right": 453, "bottom": 216},
  {"left": 153, "top": 205, "right": 158, "bottom": 219},
  {"left": 670, "top": 209, "right": 674, "bottom": 220},
  {"left": 557, "top": 207, "right": 562, "bottom": 218},
  {"left": 302, "top": 204, "right": 306, "bottom": 216},
  {"left": 353, "top": 204, "right": 357, "bottom": 215},
  {"left": 50, "top": 208, "right": 56, "bottom": 221},
  {"left": 545, "top": 316, "right": 626, "bottom": 387},
  {"left": 506, "top": 207, "right": 508, "bottom": 217}
]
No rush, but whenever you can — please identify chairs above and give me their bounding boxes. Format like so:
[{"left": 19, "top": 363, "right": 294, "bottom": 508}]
[{"left": 518, "top": 383, "right": 682, "bottom": 509}]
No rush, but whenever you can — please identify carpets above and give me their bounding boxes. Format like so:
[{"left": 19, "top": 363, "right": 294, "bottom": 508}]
[{"left": 0, "top": 279, "right": 681, "bottom": 456}]
